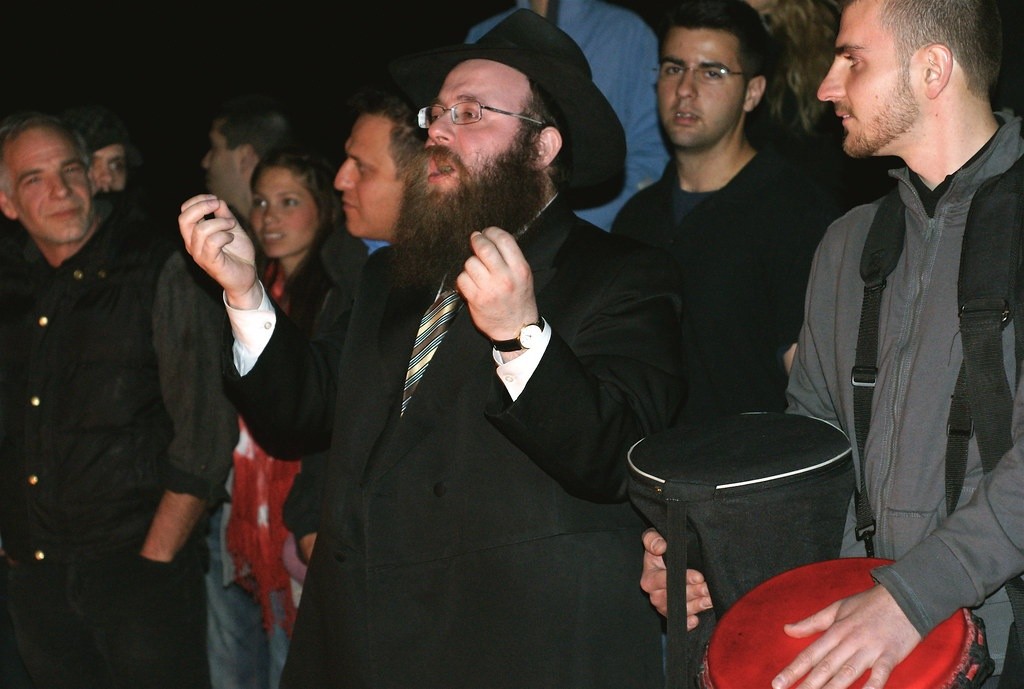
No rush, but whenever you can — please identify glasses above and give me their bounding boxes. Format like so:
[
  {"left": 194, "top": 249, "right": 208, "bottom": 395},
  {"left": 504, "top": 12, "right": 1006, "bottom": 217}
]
[
  {"left": 657, "top": 63, "right": 743, "bottom": 84},
  {"left": 418, "top": 100, "right": 544, "bottom": 129}
]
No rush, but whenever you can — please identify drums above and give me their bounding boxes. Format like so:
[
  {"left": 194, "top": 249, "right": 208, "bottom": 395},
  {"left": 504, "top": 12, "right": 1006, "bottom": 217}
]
[{"left": 698, "top": 557, "right": 995, "bottom": 689}]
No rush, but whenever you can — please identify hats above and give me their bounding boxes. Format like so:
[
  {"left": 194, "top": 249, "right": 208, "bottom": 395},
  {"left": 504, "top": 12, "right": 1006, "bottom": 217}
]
[
  {"left": 60, "top": 106, "right": 142, "bottom": 172},
  {"left": 463, "top": 11, "right": 627, "bottom": 211}
]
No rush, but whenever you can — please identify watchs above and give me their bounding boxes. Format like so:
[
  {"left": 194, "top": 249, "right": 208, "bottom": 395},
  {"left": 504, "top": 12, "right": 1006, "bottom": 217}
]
[{"left": 492, "top": 314, "right": 545, "bottom": 352}]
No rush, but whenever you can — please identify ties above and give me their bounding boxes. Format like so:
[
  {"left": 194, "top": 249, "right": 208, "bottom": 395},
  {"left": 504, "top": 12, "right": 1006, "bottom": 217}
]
[{"left": 400, "top": 288, "right": 461, "bottom": 417}]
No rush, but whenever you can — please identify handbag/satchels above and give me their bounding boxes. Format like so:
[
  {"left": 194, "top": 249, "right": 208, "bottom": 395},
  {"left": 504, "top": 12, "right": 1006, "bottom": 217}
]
[{"left": 625, "top": 409, "right": 854, "bottom": 689}]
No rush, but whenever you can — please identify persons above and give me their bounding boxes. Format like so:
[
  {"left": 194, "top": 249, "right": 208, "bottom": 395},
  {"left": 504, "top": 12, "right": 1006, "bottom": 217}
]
[
  {"left": 226, "top": 148, "right": 344, "bottom": 639},
  {"left": 178, "top": 7, "right": 682, "bottom": 689},
  {"left": 611, "top": 0, "right": 827, "bottom": 418},
  {"left": 0, "top": 106, "right": 240, "bottom": 689},
  {"left": 333, "top": 78, "right": 440, "bottom": 245},
  {"left": 640, "top": 0, "right": 1024, "bottom": 689},
  {"left": 467, "top": 0, "right": 671, "bottom": 219},
  {"left": 200, "top": 104, "right": 295, "bottom": 689}
]
